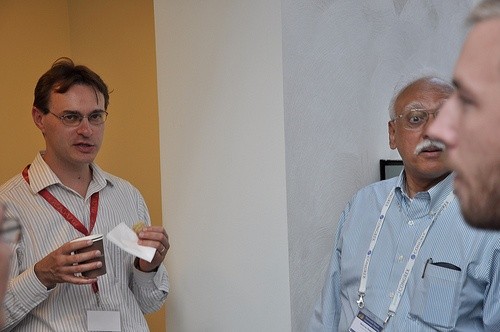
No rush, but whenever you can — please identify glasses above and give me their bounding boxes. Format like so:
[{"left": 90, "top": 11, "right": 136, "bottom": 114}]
[
  {"left": 393, "top": 109, "right": 441, "bottom": 130},
  {"left": 49, "top": 110, "right": 108, "bottom": 126}
]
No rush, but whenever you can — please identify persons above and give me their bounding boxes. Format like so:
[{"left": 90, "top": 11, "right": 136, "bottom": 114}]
[
  {"left": 426, "top": 0, "right": 500, "bottom": 230},
  {"left": 311, "top": 77, "right": 500, "bottom": 332},
  {"left": 0, "top": 64, "right": 170, "bottom": 332}
]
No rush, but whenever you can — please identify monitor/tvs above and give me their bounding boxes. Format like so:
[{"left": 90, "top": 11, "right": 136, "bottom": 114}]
[{"left": 380, "top": 159, "right": 403, "bottom": 180}]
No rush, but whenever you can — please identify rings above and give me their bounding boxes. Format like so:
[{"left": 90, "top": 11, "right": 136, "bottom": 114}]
[{"left": 160, "top": 246, "right": 165, "bottom": 254}]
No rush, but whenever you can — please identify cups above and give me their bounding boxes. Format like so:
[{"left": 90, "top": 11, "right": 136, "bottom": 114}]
[{"left": 70, "top": 234, "right": 107, "bottom": 279}]
